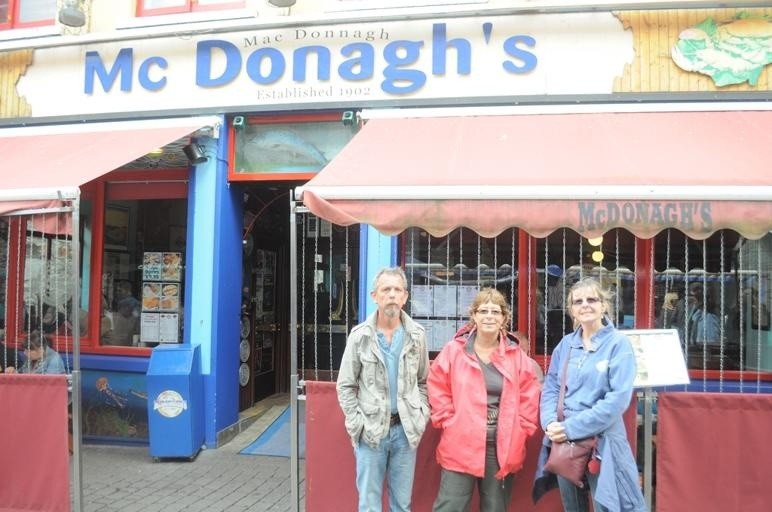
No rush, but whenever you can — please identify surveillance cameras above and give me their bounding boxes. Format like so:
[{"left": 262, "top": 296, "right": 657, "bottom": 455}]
[{"left": 182, "top": 143, "right": 208, "bottom": 166}]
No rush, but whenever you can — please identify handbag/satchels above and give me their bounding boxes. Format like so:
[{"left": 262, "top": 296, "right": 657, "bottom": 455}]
[{"left": 541, "top": 431, "right": 600, "bottom": 489}]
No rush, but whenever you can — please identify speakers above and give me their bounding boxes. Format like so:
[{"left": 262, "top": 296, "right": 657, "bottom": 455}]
[
  {"left": 342, "top": 111, "right": 356, "bottom": 127},
  {"left": 232, "top": 116, "right": 246, "bottom": 130}
]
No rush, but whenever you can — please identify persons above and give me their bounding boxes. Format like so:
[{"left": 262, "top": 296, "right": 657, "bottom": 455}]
[
  {"left": 335, "top": 266, "right": 432, "bottom": 512},
  {"left": 424, "top": 284, "right": 544, "bottom": 511},
  {"left": 21, "top": 277, "right": 143, "bottom": 346},
  {"left": 652, "top": 281, "right": 771, "bottom": 372},
  {"left": 530, "top": 267, "right": 651, "bottom": 512},
  {"left": 5, "top": 331, "right": 68, "bottom": 376}
]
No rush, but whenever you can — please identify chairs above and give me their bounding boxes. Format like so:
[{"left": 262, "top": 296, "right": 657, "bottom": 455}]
[{"left": 63, "top": 370, "right": 72, "bottom": 448}]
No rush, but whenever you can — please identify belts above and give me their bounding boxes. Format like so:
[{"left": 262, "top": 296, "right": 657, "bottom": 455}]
[{"left": 387, "top": 412, "right": 401, "bottom": 427}]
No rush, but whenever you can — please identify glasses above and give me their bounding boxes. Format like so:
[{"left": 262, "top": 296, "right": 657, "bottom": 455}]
[
  {"left": 571, "top": 297, "right": 602, "bottom": 305},
  {"left": 475, "top": 308, "right": 503, "bottom": 315}
]
